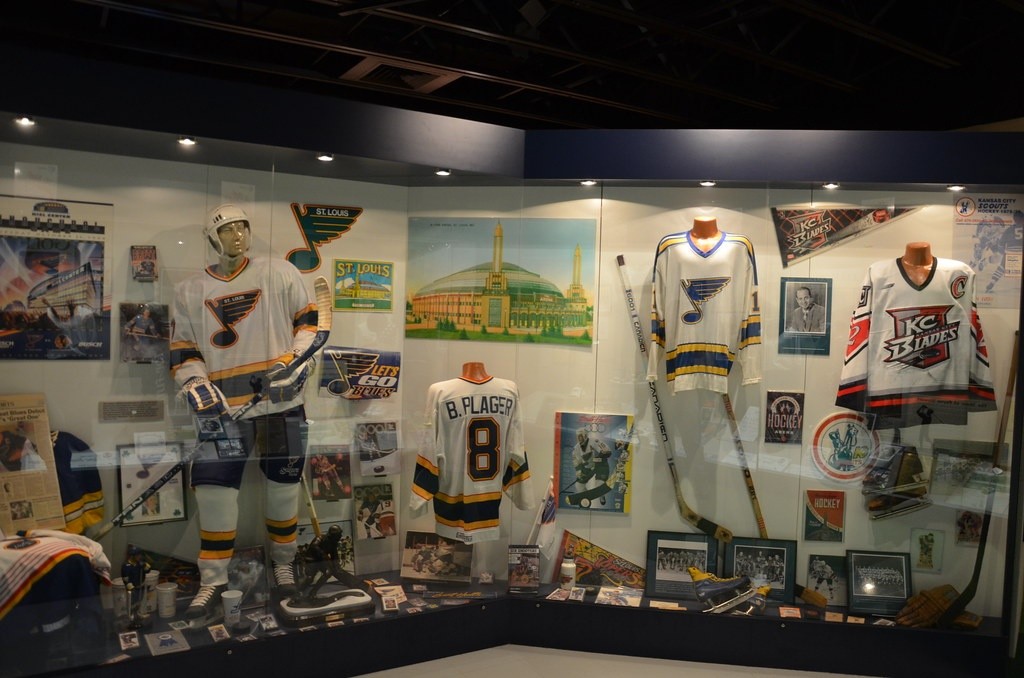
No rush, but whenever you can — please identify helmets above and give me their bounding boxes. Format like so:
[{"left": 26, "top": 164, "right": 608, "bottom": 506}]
[
  {"left": 575, "top": 428, "right": 589, "bottom": 446},
  {"left": 202, "top": 201, "right": 251, "bottom": 255}
]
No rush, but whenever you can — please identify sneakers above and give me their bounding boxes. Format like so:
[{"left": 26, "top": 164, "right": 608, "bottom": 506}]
[
  {"left": 688, "top": 566, "right": 749, "bottom": 606},
  {"left": 269, "top": 558, "right": 298, "bottom": 603},
  {"left": 184, "top": 584, "right": 229, "bottom": 629},
  {"left": 725, "top": 582, "right": 771, "bottom": 616}
]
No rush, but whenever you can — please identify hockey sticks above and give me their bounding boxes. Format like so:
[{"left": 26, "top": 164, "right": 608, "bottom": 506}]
[
  {"left": 719, "top": 393, "right": 828, "bottom": 609},
  {"left": 930, "top": 328, "right": 1021, "bottom": 632},
  {"left": 560, "top": 475, "right": 586, "bottom": 496},
  {"left": 564, "top": 422, "right": 638, "bottom": 508},
  {"left": 92, "top": 274, "right": 336, "bottom": 545},
  {"left": 616, "top": 250, "right": 736, "bottom": 545}
]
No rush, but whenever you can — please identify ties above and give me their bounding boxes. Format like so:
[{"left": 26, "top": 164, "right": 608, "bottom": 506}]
[{"left": 803, "top": 312, "right": 808, "bottom": 328}]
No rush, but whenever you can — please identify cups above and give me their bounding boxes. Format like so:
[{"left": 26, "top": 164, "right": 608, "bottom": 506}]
[
  {"left": 111, "top": 577, "right": 128, "bottom": 617},
  {"left": 221, "top": 590, "right": 244, "bottom": 627},
  {"left": 156, "top": 582, "right": 178, "bottom": 618},
  {"left": 138, "top": 569, "right": 160, "bottom": 613}
]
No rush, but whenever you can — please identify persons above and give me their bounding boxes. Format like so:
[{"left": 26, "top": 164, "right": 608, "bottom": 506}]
[
  {"left": 810, "top": 557, "right": 841, "bottom": 599},
  {"left": 736, "top": 550, "right": 784, "bottom": 584},
  {"left": 123, "top": 302, "right": 160, "bottom": 355},
  {"left": 790, "top": 287, "right": 825, "bottom": 333},
  {"left": 572, "top": 428, "right": 612, "bottom": 505},
  {"left": 917, "top": 533, "right": 935, "bottom": 568},
  {"left": 357, "top": 491, "right": 396, "bottom": 540},
  {"left": 855, "top": 566, "right": 904, "bottom": 587},
  {"left": 835, "top": 242, "right": 997, "bottom": 429},
  {"left": 170, "top": 202, "right": 318, "bottom": 616},
  {"left": 289, "top": 524, "right": 344, "bottom": 606},
  {"left": 412, "top": 544, "right": 457, "bottom": 575},
  {"left": 658, "top": 549, "right": 706, "bottom": 575},
  {"left": 311, "top": 451, "right": 345, "bottom": 494},
  {"left": 122, "top": 546, "right": 150, "bottom": 629},
  {"left": 410, "top": 363, "right": 535, "bottom": 543},
  {"left": 970, "top": 208, "right": 1024, "bottom": 292},
  {"left": 646, "top": 216, "right": 762, "bottom": 394}
]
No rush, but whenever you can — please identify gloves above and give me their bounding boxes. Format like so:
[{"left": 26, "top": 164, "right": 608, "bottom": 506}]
[
  {"left": 172, "top": 358, "right": 232, "bottom": 428},
  {"left": 594, "top": 454, "right": 605, "bottom": 468},
  {"left": 268, "top": 351, "right": 316, "bottom": 404},
  {"left": 576, "top": 470, "right": 587, "bottom": 481},
  {"left": 896, "top": 584, "right": 961, "bottom": 628}
]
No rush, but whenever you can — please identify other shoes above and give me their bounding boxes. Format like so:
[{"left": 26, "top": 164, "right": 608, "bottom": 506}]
[
  {"left": 860, "top": 442, "right": 925, "bottom": 491},
  {"left": 867, "top": 485, "right": 929, "bottom": 516},
  {"left": 599, "top": 496, "right": 607, "bottom": 504}
]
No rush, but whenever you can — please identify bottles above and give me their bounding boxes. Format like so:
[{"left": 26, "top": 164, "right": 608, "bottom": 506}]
[{"left": 560, "top": 555, "right": 576, "bottom": 590}]
